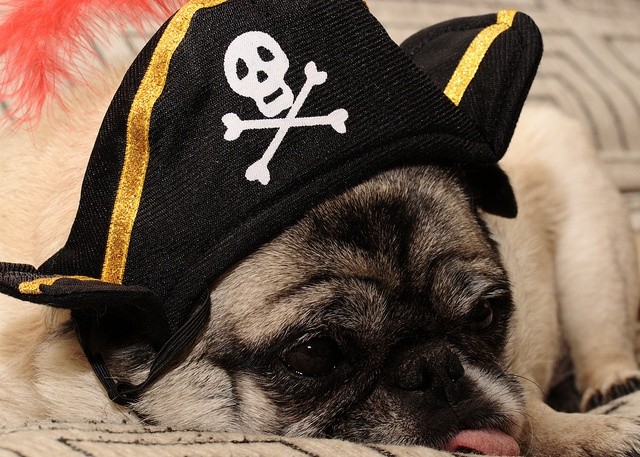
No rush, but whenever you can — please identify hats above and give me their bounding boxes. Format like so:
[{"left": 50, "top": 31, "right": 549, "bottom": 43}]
[{"left": 0, "top": 0, "right": 542, "bottom": 406}]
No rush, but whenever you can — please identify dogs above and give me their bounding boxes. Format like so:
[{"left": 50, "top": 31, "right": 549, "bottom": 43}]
[{"left": 0, "top": 0, "right": 640, "bottom": 457}]
